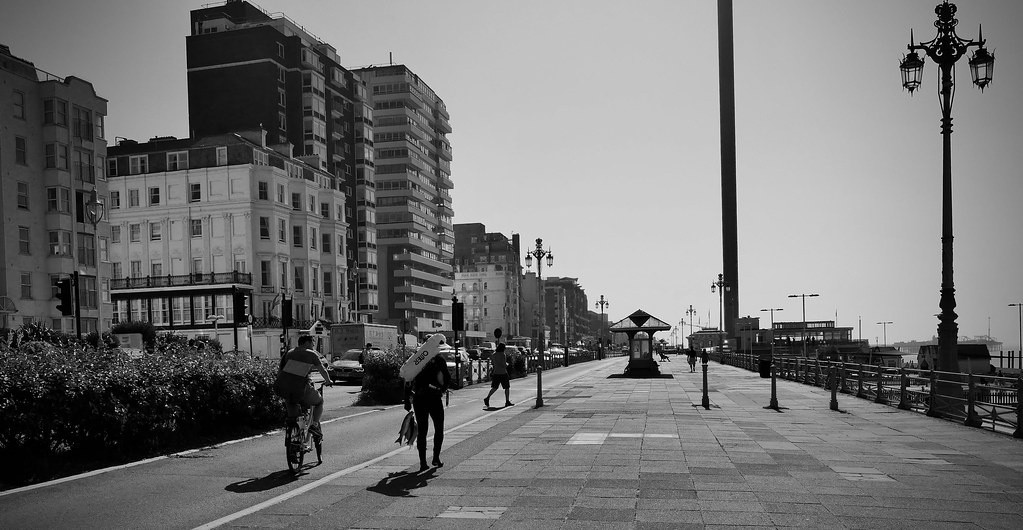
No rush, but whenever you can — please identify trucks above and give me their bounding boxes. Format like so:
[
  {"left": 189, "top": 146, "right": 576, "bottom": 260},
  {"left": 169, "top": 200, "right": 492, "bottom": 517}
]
[{"left": 329, "top": 322, "right": 418, "bottom": 364}]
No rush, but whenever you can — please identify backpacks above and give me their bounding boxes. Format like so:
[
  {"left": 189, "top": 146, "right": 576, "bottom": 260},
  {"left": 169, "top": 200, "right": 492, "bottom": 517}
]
[{"left": 358, "top": 349, "right": 367, "bottom": 364}]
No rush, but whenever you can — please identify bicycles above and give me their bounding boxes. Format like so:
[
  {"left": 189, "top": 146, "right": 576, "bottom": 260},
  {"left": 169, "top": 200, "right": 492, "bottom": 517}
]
[{"left": 271, "top": 379, "right": 335, "bottom": 475}]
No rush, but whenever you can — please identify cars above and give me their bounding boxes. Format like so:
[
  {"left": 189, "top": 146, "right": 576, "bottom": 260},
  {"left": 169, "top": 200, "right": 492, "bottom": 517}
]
[
  {"left": 436, "top": 338, "right": 590, "bottom": 388},
  {"left": 326, "top": 348, "right": 385, "bottom": 386}
]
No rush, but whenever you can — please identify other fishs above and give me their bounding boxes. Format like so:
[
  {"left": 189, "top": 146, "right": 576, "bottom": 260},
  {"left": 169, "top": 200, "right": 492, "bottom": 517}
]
[{"left": 395, "top": 411, "right": 418, "bottom": 446}]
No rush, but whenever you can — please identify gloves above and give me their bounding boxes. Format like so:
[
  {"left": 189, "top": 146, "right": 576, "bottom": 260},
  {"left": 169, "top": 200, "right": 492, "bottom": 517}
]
[
  {"left": 440, "top": 385, "right": 448, "bottom": 394},
  {"left": 404, "top": 403, "right": 411, "bottom": 412}
]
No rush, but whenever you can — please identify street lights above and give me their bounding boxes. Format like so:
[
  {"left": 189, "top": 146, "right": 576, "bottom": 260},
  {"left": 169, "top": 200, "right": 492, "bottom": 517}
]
[
  {"left": 686, "top": 305, "right": 697, "bottom": 351},
  {"left": 1008, "top": 303, "right": 1023, "bottom": 369},
  {"left": 670, "top": 326, "right": 679, "bottom": 354},
  {"left": 876, "top": 321, "right": 893, "bottom": 347},
  {"left": 898, "top": 1, "right": 997, "bottom": 422},
  {"left": 760, "top": 308, "right": 784, "bottom": 362},
  {"left": 710, "top": 273, "right": 731, "bottom": 364},
  {"left": 207, "top": 314, "right": 224, "bottom": 340},
  {"left": 788, "top": 294, "right": 820, "bottom": 357},
  {"left": 524, "top": 237, "right": 554, "bottom": 408},
  {"left": 595, "top": 295, "right": 609, "bottom": 360},
  {"left": 84, "top": 186, "right": 105, "bottom": 348},
  {"left": 678, "top": 317, "right": 686, "bottom": 355}
]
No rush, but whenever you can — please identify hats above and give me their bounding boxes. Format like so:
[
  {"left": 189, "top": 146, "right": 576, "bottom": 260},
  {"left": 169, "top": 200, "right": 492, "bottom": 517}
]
[
  {"left": 499, "top": 343, "right": 506, "bottom": 349},
  {"left": 424, "top": 334, "right": 433, "bottom": 340}
]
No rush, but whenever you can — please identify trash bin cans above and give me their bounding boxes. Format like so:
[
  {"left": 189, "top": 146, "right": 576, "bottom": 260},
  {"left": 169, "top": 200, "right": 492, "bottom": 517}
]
[{"left": 759, "top": 354, "right": 771, "bottom": 379}]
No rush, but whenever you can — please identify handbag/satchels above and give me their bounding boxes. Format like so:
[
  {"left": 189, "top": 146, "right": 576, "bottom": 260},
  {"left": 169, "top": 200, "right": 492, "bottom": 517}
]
[{"left": 273, "top": 371, "right": 308, "bottom": 401}]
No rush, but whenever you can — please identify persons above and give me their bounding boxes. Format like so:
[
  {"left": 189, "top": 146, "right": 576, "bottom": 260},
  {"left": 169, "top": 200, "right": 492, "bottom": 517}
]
[
  {"left": 273, "top": 335, "right": 333, "bottom": 439},
  {"left": 687, "top": 346, "right": 697, "bottom": 373},
  {"left": 699, "top": 349, "right": 710, "bottom": 364},
  {"left": 361, "top": 343, "right": 373, "bottom": 368},
  {"left": 484, "top": 342, "right": 515, "bottom": 408},
  {"left": 658, "top": 352, "right": 671, "bottom": 362},
  {"left": 403, "top": 334, "right": 452, "bottom": 471}
]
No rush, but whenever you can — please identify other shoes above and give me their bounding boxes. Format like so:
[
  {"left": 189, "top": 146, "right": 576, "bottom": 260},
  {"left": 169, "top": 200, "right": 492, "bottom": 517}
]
[
  {"left": 484, "top": 397, "right": 489, "bottom": 407},
  {"left": 432, "top": 457, "right": 443, "bottom": 467},
  {"left": 420, "top": 462, "right": 429, "bottom": 471},
  {"left": 505, "top": 401, "right": 514, "bottom": 407},
  {"left": 308, "top": 425, "right": 323, "bottom": 437},
  {"left": 290, "top": 452, "right": 298, "bottom": 464}
]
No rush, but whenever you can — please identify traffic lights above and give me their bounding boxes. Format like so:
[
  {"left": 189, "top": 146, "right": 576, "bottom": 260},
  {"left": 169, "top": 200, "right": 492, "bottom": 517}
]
[{"left": 54, "top": 278, "right": 73, "bottom": 316}]
